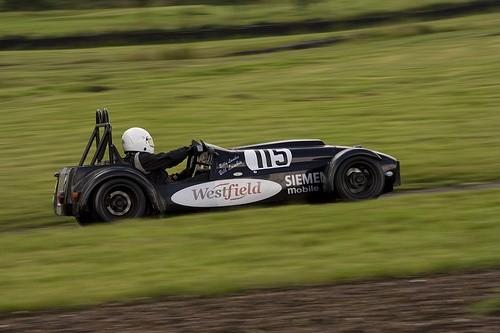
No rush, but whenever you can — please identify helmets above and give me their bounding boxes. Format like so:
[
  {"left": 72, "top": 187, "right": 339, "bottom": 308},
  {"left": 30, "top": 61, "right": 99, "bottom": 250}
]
[{"left": 121, "top": 127, "right": 155, "bottom": 155}]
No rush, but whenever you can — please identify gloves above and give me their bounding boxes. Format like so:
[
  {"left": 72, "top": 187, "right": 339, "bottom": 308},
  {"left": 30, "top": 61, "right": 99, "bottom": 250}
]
[
  {"left": 183, "top": 142, "right": 204, "bottom": 156},
  {"left": 172, "top": 167, "right": 192, "bottom": 181}
]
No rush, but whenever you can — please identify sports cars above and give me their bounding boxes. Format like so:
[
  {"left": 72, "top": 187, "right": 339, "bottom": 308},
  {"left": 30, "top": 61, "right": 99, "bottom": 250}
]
[{"left": 53, "top": 107, "right": 404, "bottom": 226}]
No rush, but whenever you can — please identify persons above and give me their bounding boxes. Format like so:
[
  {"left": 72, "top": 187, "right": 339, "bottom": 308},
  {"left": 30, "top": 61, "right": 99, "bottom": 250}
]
[{"left": 121, "top": 126, "right": 210, "bottom": 192}]
